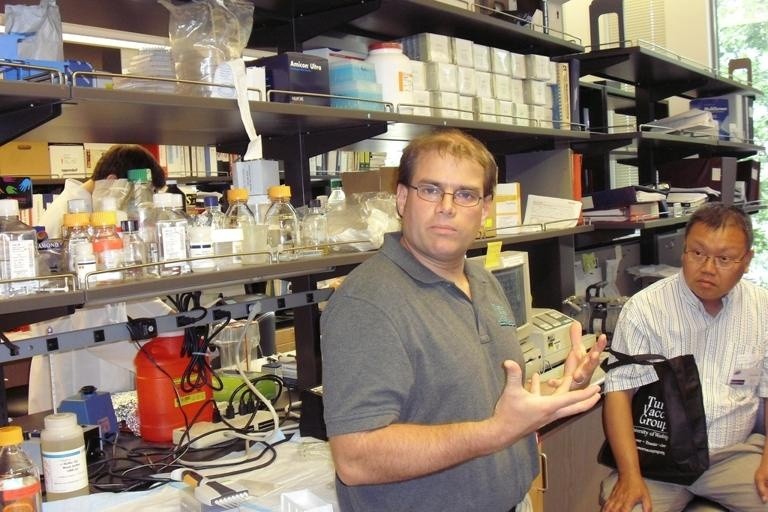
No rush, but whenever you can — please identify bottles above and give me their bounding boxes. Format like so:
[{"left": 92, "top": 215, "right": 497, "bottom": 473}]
[
  {"left": 0, "top": 167, "right": 348, "bottom": 291},
  {"left": 0, "top": 413, "right": 90, "bottom": 512}
]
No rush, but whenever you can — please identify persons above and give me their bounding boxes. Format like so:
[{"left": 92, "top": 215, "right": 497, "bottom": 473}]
[
  {"left": 600, "top": 201, "right": 768, "bottom": 512},
  {"left": 318, "top": 128, "right": 608, "bottom": 512},
  {"left": 27, "top": 143, "right": 181, "bottom": 415}
]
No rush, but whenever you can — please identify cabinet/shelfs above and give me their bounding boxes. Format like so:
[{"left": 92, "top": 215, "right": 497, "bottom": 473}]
[{"left": 0, "top": 1, "right": 767, "bottom": 512}]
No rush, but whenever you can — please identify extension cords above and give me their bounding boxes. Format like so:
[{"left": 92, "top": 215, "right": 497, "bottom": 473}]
[{"left": 171, "top": 407, "right": 277, "bottom": 450}]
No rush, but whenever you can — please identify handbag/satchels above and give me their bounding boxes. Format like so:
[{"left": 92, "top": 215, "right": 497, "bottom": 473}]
[{"left": 597, "top": 348, "right": 711, "bottom": 487}]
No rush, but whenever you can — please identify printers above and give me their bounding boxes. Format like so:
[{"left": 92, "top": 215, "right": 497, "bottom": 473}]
[{"left": 532, "top": 307, "right": 582, "bottom": 371}]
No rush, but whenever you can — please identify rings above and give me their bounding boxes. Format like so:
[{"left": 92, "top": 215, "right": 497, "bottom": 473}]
[{"left": 577, "top": 379, "right": 585, "bottom": 386}]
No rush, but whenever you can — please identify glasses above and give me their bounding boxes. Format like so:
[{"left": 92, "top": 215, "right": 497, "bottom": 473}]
[
  {"left": 682, "top": 244, "right": 748, "bottom": 269},
  {"left": 396, "top": 180, "right": 486, "bottom": 207}
]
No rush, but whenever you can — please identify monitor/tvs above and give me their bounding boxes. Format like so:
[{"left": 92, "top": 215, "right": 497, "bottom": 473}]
[{"left": 466, "top": 250, "right": 533, "bottom": 359}]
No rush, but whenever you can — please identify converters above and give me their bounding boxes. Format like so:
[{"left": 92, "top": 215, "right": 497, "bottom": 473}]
[{"left": 128, "top": 317, "right": 158, "bottom": 341}]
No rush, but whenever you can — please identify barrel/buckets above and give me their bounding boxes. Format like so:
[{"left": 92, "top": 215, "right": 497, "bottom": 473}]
[
  {"left": 134, "top": 337, "right": 213, "bottom": 442},
  {"left": 364, "top": 43, "right": 416, "bottom": 113}
]
